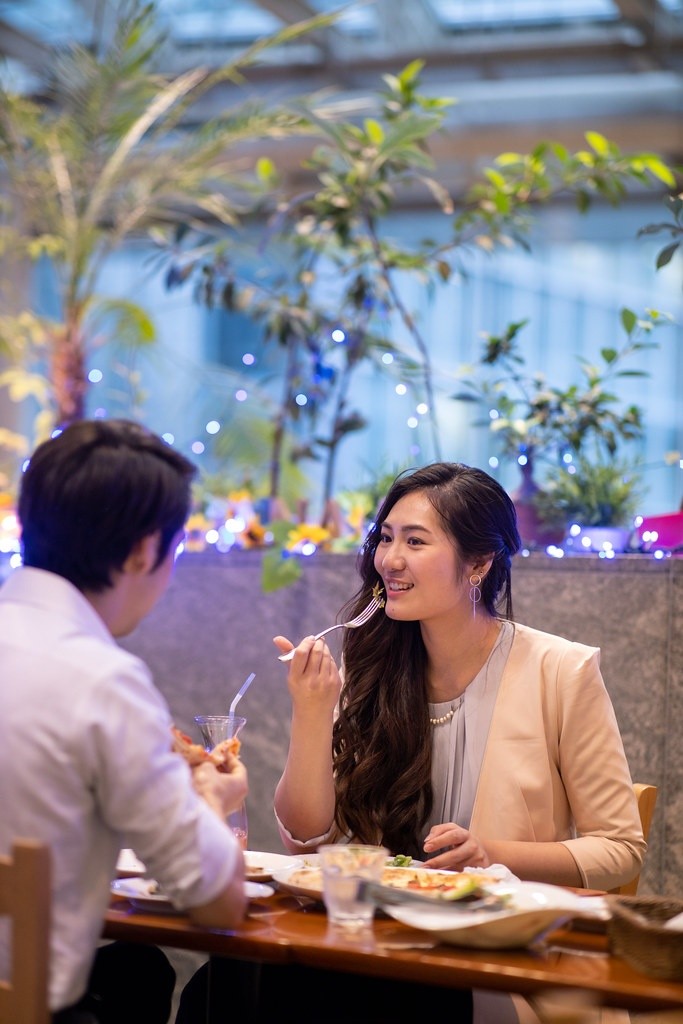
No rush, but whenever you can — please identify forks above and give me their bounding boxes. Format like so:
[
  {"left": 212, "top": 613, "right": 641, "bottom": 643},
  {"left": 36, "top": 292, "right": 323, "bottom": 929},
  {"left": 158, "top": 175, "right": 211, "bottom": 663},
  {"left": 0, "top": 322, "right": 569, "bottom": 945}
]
[{"left": 277, "top": 596, "right": 381, "bottom": 662}]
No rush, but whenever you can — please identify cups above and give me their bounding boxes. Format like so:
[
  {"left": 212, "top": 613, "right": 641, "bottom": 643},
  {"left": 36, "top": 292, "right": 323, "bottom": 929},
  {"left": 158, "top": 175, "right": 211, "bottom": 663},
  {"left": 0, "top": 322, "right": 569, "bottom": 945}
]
[{"left": 316, "top": 844, "right": 390, "bottom": 927}]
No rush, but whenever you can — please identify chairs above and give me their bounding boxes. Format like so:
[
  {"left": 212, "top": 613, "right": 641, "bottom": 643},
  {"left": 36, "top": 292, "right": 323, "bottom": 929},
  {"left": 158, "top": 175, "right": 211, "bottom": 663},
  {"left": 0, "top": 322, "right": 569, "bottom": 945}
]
[
  {"left": 605, "top": 782, "right": 658, "bottom": 896},
  {"left": 0, "top": 838, "right": 54, "bottom": 1024}
]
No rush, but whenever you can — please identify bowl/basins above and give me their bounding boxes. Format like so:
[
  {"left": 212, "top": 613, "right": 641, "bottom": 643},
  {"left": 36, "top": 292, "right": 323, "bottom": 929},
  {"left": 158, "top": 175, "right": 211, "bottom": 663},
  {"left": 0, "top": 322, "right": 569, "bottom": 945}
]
[{"left": 381, "top": 881, "right": 588, "bottom": 949}]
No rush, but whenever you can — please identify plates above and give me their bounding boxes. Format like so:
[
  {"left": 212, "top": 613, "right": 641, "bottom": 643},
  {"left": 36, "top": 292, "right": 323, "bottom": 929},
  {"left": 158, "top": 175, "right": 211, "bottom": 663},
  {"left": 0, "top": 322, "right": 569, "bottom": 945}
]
[
  {"left": 117, "top": 848, "right": 147, "bottom": 876},
  {"left": 384, "top": 856, "right": 424, "bottom": 869},
  {"left": 272, "top": 854, "right": 323, "bottom": 900},
  {"left": 242, "top": 851, "right": 305, "bottom": 883},
  {"left": 110, "top": 877, "right": 275, "bottom": 915}
]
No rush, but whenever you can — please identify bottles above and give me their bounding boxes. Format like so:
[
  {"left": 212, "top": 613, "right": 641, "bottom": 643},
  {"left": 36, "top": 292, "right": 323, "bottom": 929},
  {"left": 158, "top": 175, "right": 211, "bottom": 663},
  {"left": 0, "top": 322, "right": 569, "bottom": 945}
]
[{"left": 193, "top": 715, "right": 248, "bottom": 851}]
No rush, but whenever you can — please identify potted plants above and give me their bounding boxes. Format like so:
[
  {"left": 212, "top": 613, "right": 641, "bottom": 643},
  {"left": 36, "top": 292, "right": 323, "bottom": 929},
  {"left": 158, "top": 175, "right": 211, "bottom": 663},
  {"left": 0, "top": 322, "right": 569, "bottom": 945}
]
[{"left": 451, "top": 304, "right": 663, "bottom": 552}]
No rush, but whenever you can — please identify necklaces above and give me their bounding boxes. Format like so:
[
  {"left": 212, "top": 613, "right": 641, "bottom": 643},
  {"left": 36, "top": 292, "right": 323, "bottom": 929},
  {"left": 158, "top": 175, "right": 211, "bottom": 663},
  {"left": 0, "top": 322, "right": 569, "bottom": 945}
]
[{"left": 429, "top": 710, "right": 454, "bottom": 725}]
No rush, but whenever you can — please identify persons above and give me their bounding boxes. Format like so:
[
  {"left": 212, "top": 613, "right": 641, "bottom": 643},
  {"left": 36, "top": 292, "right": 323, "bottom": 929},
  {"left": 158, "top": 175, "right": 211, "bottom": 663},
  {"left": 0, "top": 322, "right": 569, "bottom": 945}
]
[
  {"left": 172, "top": 462, "right": 649, "bottom": 1024},
  {"left": 1, "top": 418, "right": 251, "bottom": 1024}
]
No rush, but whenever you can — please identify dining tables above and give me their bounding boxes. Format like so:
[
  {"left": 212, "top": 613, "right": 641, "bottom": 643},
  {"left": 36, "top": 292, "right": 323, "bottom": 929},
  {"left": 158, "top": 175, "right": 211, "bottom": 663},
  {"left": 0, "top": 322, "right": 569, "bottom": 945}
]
[{"left": 97, "top": 884, "right": 683, "bottom": 1024}]
